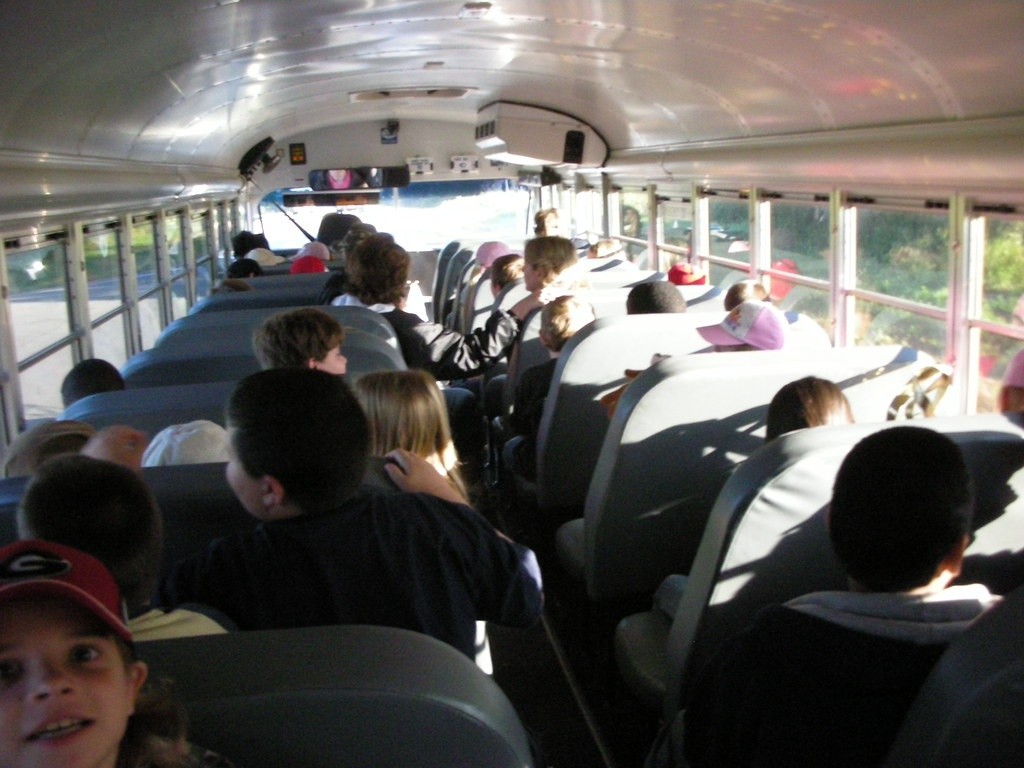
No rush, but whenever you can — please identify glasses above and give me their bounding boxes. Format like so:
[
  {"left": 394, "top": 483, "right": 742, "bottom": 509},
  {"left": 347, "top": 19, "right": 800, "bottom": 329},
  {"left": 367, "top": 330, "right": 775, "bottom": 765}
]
[{"left": 532, "top": 226, "right": 543, "bottom": 233}]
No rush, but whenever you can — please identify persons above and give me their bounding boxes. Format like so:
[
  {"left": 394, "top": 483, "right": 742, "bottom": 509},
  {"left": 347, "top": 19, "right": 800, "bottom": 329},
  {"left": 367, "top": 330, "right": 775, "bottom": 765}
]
[
  {"left": 641, "top": 427, "right": 1007, "bottom": 767},
  {"left": 287, "top": 241, "right": 335, "bottom": 274},
  {"left": 725, "top": 280, "right": 772, "bottom": 314},
  {"left": 313, "top": 169, "right": 369, "bottom": 190},
  {"left": 446, "top": 207, "right": 629, "bottom": 301},
  {"left": 0, "top": 540, "right": 232, "bottom": 767},
  {"left": 669, "top": 260, "right": 706, "bottom": 287},
  {"left": 61, "top": 359, "right": 127, "bottom": 411},
  {"left": 15, "top": 455, "right": 167, "bottom": 617},
  {"left": 599, "top": 368, "right": 647, "bottom": 423},
  {"left": 0, "top": 368, "right": 545, "bottom": 683},
  {"left": 228, "top": 259, "right": 264, "bottom": 279},
  {"left": 627, "top": 281, "right": 688, "bottom": 314},
  {"left": 250, "top": 307, "right": 347, "bottom": 375},
  {"left": 696, "top": 299, "right": 791, "bottom": 352},
  {"left": 317, "top": 213, "right": 378, "bottom": 263},
  {"left": 317, "top": 232, "right": 562, "bottom": 467},
  {"left": 998, "top": 348, "right": 1024, "bottom": 430},
  {"left": 501, "top": 296, "right": 597, "bottom": 481},
  {"left": 651, "top": 376, "right": 856, "bottom": 633},
  {"left": 230, "top": 228, "right": 287, "bottom": 267}
]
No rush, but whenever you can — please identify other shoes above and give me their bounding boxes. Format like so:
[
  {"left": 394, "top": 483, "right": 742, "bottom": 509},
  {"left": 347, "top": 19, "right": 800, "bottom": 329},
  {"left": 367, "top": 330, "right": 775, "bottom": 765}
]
[{"left": 471, "top": 481, "right": 505, "bottom": 512}]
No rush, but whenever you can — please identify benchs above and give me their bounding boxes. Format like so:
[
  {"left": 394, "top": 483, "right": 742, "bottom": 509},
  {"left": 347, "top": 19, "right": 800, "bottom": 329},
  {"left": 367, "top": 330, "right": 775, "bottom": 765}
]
[{"left": 0, "top": 241, "right": 1024, "bottom": 768}]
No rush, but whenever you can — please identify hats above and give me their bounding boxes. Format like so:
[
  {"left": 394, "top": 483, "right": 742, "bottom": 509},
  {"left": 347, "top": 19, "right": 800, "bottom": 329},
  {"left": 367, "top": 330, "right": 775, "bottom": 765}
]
[
  {"left": 141, "top": 419, "right": 227, "bottom": 467},
  {"left": 696, "top": 299, "right": 790, "bottom": 349},
  {"left": 0, "top": 540, "right": 133, "bottom": 640},
  {"left": 2, "top": 420, "right": 93, "bottom": 478},
  {"left": 287, "top": 242, "right": 331, "bottom": 261},
  {"left": 479, "top": 241, "right": 520, "bottom": 267},
  {"left": 771, "top": 260, "right": 800, "bottom": 295},
  {"left": 244, "top": 248, "right": 285, "bottom": 264},
  {"left": 668, "top": 263, "right": 706, "bottom": 285},
  {"left": 290, "top": 255, "right": 325, "bottom": 275}
]
